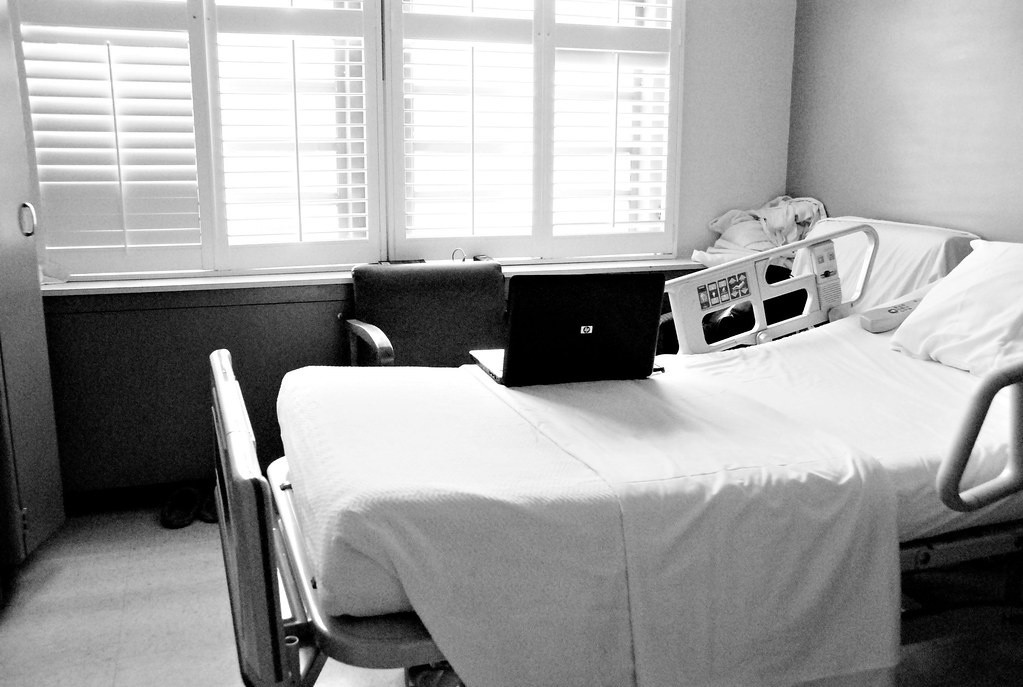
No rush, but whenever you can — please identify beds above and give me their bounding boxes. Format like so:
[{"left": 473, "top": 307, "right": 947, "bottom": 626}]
[{"left": 207, "top": 236, "right": 1023, "bottom": 687}]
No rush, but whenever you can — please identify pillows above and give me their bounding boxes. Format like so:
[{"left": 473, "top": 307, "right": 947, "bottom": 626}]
[{"left": 888, "top": 239, "right": 1023, "bottom": 377}]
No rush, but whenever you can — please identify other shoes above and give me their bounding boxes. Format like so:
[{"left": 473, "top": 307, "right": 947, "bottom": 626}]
[{"left": 159, "top": 482, "right": 219, "bottom": 529}]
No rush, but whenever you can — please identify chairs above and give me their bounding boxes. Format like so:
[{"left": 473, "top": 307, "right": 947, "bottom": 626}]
[
  {"left": 343, "top": 261, "right": 509, "bottom": 366},
  {"left": 790, "top": 216, "right": 978, "bottom": 317}
]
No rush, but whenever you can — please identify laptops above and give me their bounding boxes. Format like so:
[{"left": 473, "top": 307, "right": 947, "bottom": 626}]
[{"left": 468, "top": 270, "right": 667, "bottom": 388}]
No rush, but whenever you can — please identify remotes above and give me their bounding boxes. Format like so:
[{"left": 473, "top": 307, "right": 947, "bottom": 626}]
[{"left": 859, "top": 299, "right": 921, "bottom": 333}]
